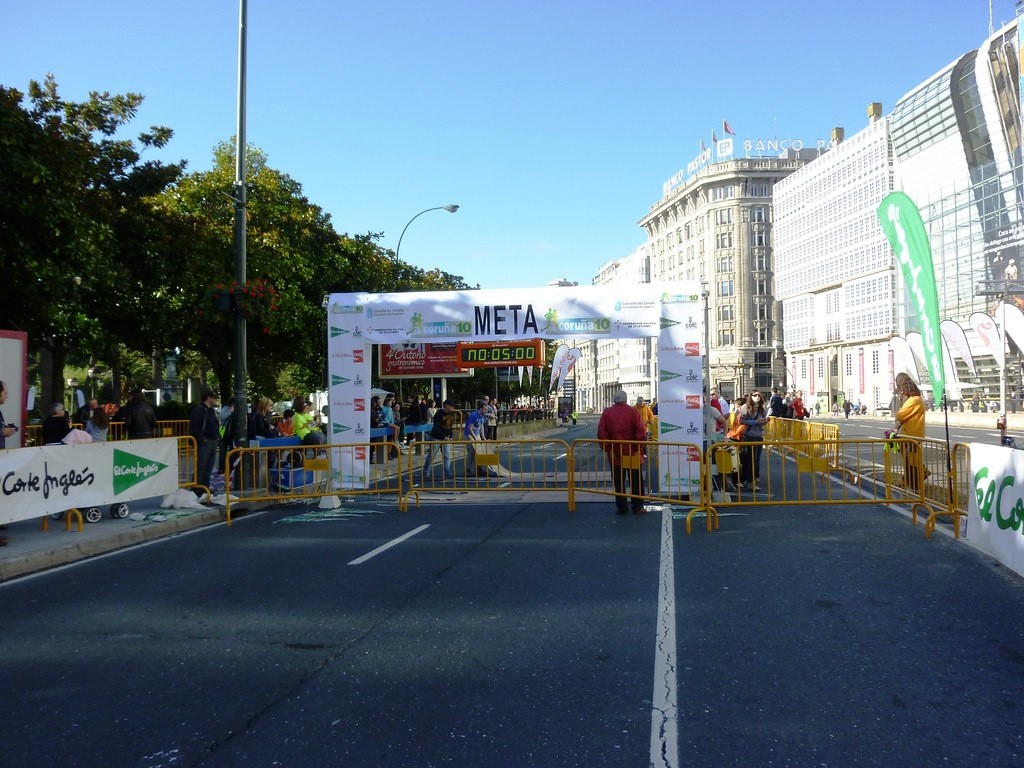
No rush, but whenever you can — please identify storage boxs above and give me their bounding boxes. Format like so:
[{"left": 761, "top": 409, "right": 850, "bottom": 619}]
[{"left": 269, "top": 463, "right": 314, "bottom": 492}]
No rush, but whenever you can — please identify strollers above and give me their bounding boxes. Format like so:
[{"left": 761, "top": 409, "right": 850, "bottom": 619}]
[{"left": 45, "top": 431, "right": 129, "bottom": 522}]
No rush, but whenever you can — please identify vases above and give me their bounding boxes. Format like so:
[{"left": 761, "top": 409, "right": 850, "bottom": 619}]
[{"left": 213, "top": 292, "right": 232, "bottom": 313}]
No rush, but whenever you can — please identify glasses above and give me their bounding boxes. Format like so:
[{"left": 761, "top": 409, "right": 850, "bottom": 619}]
[
  {"left": 269, "top": 405, "right": 273, "bottom": 406},
  {"left": 752, "top": 394, "right": 760, "bottom": 397}
]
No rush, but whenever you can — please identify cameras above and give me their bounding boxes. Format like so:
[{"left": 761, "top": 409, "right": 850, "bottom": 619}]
[{"left": 8, "top": 424, "right": 18, "bottom": 432}]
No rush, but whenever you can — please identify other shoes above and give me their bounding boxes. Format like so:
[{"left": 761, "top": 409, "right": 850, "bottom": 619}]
[
  {"left": 748, "top": 483, "right": 762, "bottom": 491},
  {"left": 895, "top": 480, "right": 909, "bottom": 489},
  {"left": 755, "top": 477, "right": 760, "bottom": 482},
  {"left": 465, "top": 469, "right": 472, "bottom": 477},
  {"left": 634, "top": 508, "right": 646, "bottom": 514},
  {"left": 370, "top": 462, "right": 375, "bottom": 464},
  {"left": 924, "top": 466, "right": 931, "bottom": 480},
  {"left": 478, "top": 470, "right": 484, "bottom": 476},
  {"left": 424, "top": 471, "right": 430, "bottom": 479},
  {"left": 846, "top": 419, "right": 848, "bottom": 421},
  {"left": 743, "top": 481, "right": 749, "bottom": 487},
  {"left": 402, "top": 446, "right": 409, "bottom": 450},
  {"left": 616, "top": 509, "right": 627, "bottom": 514}
]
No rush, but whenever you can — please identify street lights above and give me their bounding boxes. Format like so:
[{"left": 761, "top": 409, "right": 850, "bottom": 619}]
[
  {"left": 67, "top": 378, "right": 79, "bottom": 415},
  {"left": 393, "top": 205, "right": 460, "bottom": 262},
  {"left": 88, "top": 367, "right": 99, "bottom": 417}
]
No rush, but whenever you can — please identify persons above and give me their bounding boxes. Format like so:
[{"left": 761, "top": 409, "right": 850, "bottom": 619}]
[
  {"left": 423, "top": 399, "right": 456, "bottom": 480},
  {"left": 931, "top": 393, "right": 1023, "bottom": 412},
  {"left": 26, "top": 384, "right": 437, "bottom": 500},
  {"left": 462, "top": 395, "right": 546, "bottom": 477},
  {"left": 0, "top": 381, "right": 16, "bottom": 546},
  {"left": 816, "top": 399, "right": 868, "bottom": 420},
  {"left": 597, "top": 387, "right": 814, "bottom": 513},
  {"left": 893, "top": 372, "right": 931, "bottom": 493}
]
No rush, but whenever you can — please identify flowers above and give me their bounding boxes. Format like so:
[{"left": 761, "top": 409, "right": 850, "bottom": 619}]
[
  {"left": 99, "top": 402, "right": 119, "bottom": 416},
  {"left": 196, "top": 276, "right": 278, "bottom": 334}
]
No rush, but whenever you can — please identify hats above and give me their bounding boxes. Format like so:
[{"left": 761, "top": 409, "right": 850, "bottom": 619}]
[
  {"left": 613, "top": 391, "right": 627, "bottom": 402},
  {"left": 206, "top": 391, "right": 220, "bottom": 399}
]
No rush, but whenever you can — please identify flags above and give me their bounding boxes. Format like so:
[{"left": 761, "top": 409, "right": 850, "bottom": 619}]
[{"left": 701, "top": 119, "right": 734, "bottom": 151}]
